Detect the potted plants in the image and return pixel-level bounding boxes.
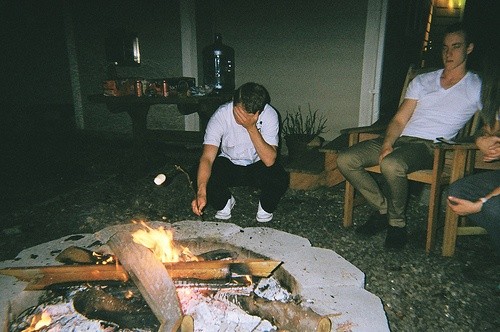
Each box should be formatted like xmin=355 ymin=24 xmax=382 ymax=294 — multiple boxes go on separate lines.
xmin=281 ymin=105 xmax=328 ymax=165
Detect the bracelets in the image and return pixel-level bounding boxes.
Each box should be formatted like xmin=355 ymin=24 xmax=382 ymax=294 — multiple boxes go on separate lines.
xmin=479 ymin=197 xmax=488 ymax=203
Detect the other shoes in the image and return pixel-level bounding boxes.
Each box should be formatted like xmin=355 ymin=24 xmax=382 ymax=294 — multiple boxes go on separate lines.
xmin=215 ymin=195 xmax=235 ymax=219
xmin=357 ymin=213 xmax=388 ymax=233
xmin=385 ymin=224 xmax=406 ymax=250
xmin=256 ymin=200 xmax=273 ymax=222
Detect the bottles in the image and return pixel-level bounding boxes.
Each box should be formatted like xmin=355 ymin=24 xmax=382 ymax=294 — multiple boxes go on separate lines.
xmin=203 ymin=33 xmax=235 ymax=94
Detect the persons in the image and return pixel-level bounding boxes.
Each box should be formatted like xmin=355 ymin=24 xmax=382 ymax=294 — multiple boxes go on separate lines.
xmin=446 ymin=107 xmax=500 ymax=248
xmin=191 ymin=83 xmax=290 ymax=222
xmin=336 ymin=20 xmax=500 ymax=250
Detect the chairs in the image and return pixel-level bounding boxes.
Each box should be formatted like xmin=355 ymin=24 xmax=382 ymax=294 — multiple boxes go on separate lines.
xmin=342 ymin=48 xmax=500 ymax=257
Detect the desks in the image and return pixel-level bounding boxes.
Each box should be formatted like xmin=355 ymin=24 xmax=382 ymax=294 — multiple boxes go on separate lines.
xmin=86 ymin=94 xmax=231 ymax=173
